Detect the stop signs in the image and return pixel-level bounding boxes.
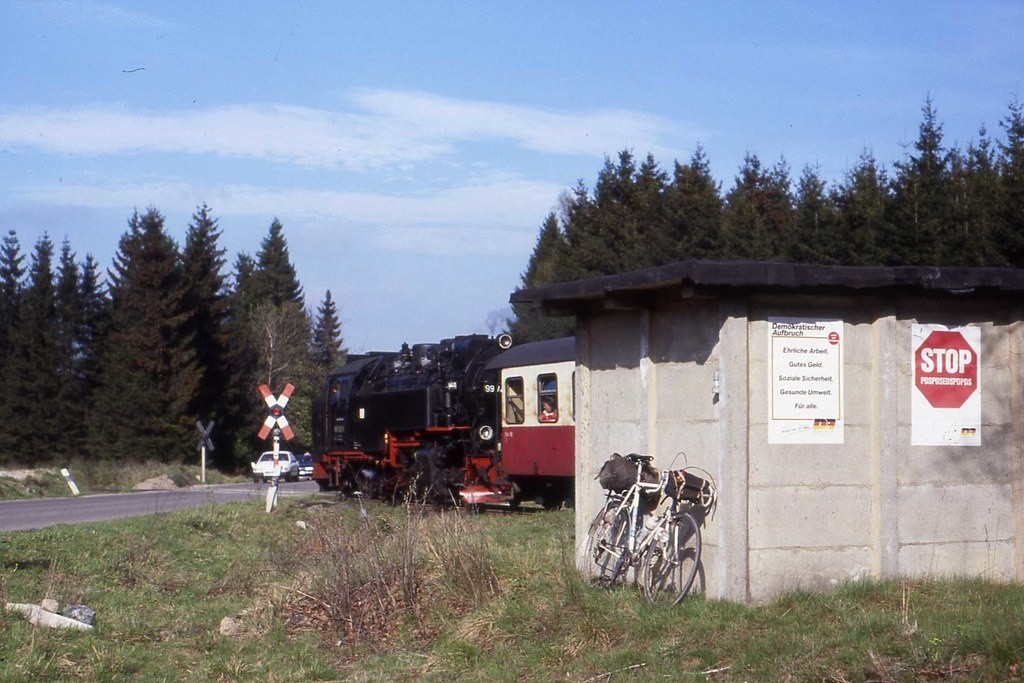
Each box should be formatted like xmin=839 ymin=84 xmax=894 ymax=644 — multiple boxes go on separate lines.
xmin=913 ymin=323 xmax=979 ymax=410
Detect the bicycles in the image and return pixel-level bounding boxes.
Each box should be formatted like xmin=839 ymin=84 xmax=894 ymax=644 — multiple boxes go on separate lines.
xmin=582 ymin=454 xmax=716 ymax=611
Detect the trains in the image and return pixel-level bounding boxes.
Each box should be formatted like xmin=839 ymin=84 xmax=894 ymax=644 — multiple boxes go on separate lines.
xmin=310 ymin=333 xmax=576 ymax=510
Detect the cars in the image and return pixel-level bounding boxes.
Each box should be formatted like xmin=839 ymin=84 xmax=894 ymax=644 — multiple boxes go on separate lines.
xmin=252 ymin=450 xmax=301 ymax=484
xmin=294 ymin=454 xmax=313 ymax=481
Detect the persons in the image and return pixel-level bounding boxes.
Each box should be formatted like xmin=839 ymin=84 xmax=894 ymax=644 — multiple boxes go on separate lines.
xmin=538 ymin=396 xmax=557 ymax=423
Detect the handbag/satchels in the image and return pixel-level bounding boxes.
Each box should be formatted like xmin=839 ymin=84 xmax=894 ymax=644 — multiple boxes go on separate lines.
xmin=665 ymin=470 xmax=714 ymax=508
xmin=594 ymin=453 xmax=661 ymax=506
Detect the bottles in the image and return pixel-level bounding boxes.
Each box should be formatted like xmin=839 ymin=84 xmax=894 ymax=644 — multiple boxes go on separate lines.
xmin=636 ymin=514 xmax=657 ymax=543
xmin=657 ymin=526 xmax=670 ymax=542
